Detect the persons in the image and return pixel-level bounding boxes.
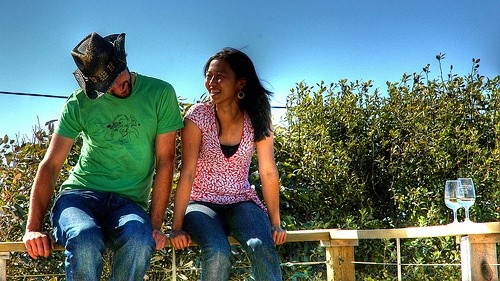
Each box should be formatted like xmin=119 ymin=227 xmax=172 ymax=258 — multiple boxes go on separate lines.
xmin=169 ymin=46 xmax=287 ymax=281
xmin=22 ymin=32 xmax=184 ymax=281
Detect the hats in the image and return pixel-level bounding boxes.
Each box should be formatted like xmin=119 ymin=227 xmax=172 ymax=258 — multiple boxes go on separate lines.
xmin=71 ymin=32 xmax=126 ymax=100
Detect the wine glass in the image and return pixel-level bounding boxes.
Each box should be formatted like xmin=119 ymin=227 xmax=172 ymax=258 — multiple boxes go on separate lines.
xmin=457 ymin=178 xmax=477 ymax=223
xmin=445 ymin=179 xmax=465 ymax=225
